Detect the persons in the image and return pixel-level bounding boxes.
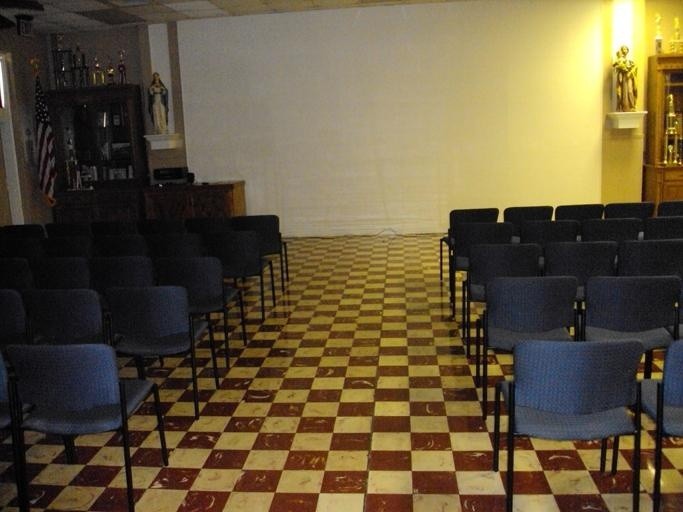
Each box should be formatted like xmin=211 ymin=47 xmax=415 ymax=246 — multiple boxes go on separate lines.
xmin=148 ymin=72 xmax=169 ymax=133
xmin=611 ymin=50 xmax=624 ymax=110
xmin=620 ymin=47 xmax=637 ymax=111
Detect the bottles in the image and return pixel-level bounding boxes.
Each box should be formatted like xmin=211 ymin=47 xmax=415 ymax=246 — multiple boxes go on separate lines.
xmin=70 ymin=49 xmax=126 ymax=88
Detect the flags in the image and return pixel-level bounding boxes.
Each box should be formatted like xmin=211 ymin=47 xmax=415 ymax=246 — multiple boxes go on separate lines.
xmin=28 ymin=69 xmax=59 ymax=208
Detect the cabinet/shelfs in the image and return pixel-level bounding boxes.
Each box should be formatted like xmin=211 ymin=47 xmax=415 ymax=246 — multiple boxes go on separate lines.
xmin=141 ymin=180 xmax=246 ymax=220
xmin=645 ymin=54 xmax=683 ymax=218
xmin=45 ymin=82 xmax=149 ymax=222
